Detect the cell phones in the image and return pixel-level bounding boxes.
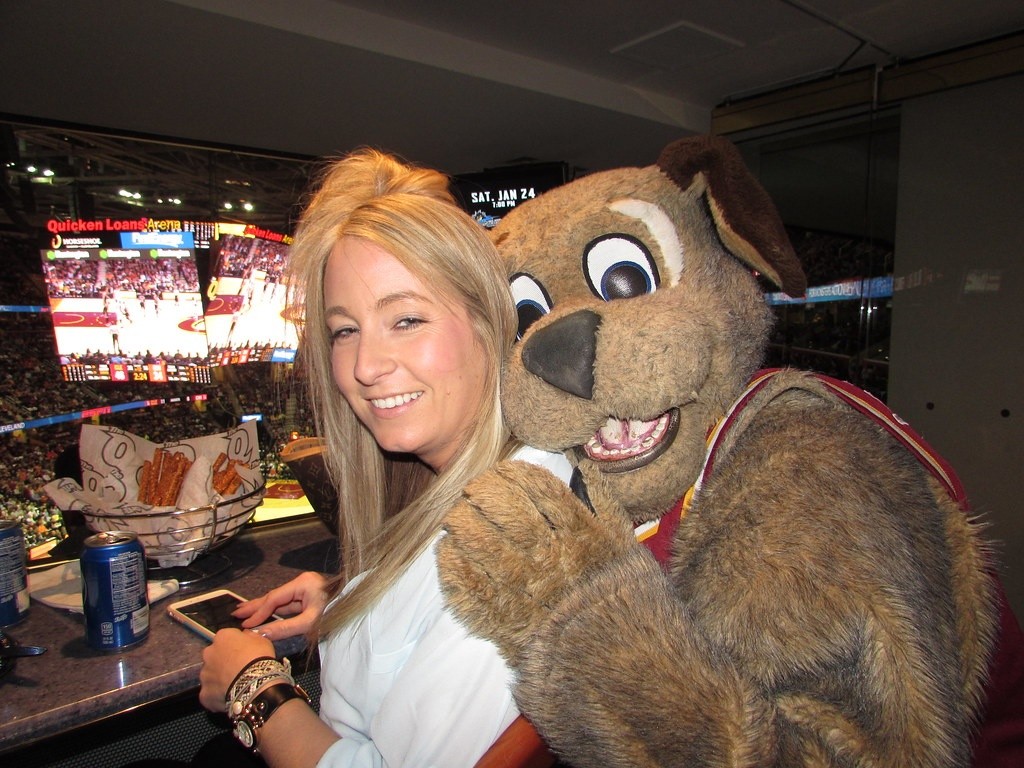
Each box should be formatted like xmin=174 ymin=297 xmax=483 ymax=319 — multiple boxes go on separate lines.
xmin=166 ymin=590 xmax=284 ymax=643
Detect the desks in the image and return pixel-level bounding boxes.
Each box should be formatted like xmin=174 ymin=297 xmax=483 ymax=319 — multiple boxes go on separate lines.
xmin=0 ymin=519 xmax=347 ymax=758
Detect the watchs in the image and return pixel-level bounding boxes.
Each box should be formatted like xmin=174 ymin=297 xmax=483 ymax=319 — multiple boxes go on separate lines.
xmin=233 ymin=684 xmax=311 ymax=753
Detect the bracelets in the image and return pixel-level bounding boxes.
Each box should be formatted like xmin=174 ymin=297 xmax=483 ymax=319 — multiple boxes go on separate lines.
xmin=225 ymin=657 xmax=295 ymax=716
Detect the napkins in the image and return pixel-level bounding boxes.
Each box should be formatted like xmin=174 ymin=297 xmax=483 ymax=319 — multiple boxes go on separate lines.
xmin=24 ymin=558 xmax=180 ymax=615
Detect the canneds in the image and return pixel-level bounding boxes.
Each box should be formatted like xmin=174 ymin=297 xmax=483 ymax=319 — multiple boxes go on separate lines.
xmin=80 ymin=530 xmax=151 ymax=651
xmin=0 ymin=519 xmax=32 ymax=629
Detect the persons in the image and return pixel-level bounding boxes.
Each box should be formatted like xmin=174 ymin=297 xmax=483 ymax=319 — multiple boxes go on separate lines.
xmin=47 ymin=257 xmax=208 ymax=366
xmin=0 ymin=283 xmax=317 ymax=548
xmin=208 ymin=233 xmax=292 ymax=351
xmin=200 ymin=152 xmax=574 ymax=768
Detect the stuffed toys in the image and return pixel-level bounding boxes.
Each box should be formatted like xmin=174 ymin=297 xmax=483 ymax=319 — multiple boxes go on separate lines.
xmin=433 ymin=134 xmax=1024 ymax=768
